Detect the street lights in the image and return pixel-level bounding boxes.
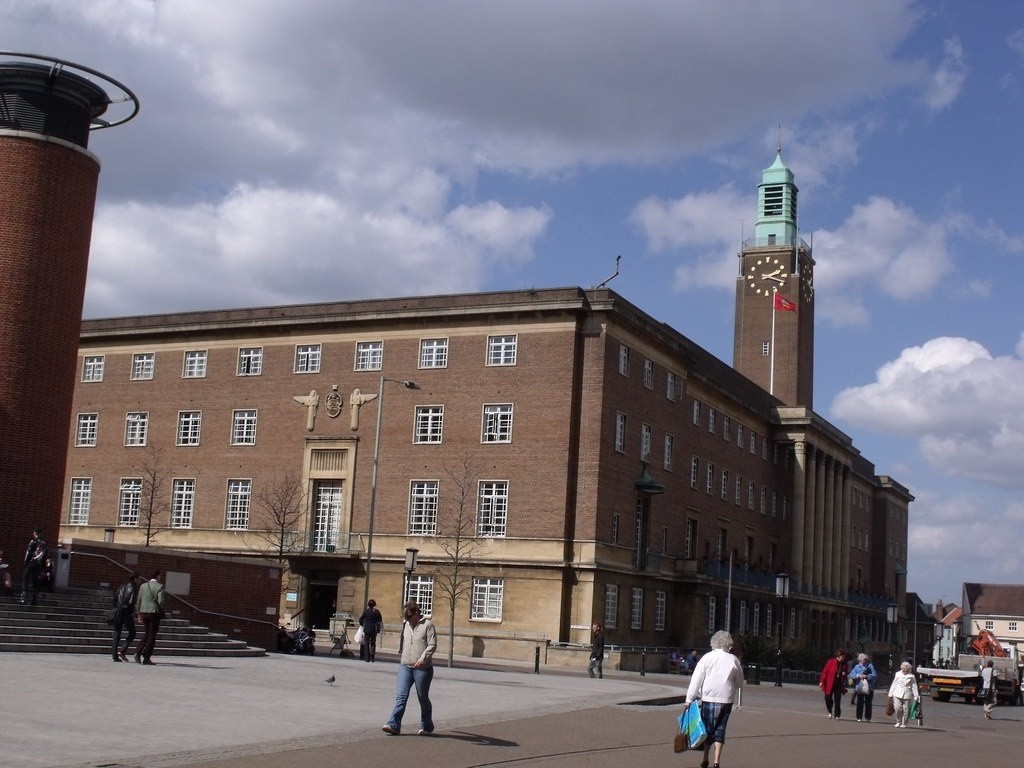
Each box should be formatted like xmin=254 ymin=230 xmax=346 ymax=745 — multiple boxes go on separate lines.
xmin=774 ymin=573 xmax=789 ymax=687
xmin=398 ymin=547 xmax=419 ymax=654
xmin=936 ymin=622 xmax=944 ymax=663
xmin=363 ymin=375 xmax=421 ymax=608
xmin=886 ymin=603 xmax=898 ymax=691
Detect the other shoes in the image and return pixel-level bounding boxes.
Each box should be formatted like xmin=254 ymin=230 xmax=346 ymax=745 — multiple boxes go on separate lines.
xmin=985 ymin=711 xmax=992 ymax=719
xmin=713 ymin=763 xmax=720 ymax=768
xmin=382 ymin=725 xmax=400 ymax=736
xmin=866 ymin=719 xmax=870 ymax=722
xmin=118 ymin=650 xmax=129 ymax=662
xmin=835 ymin=716 xmax=840 ymax=720
xmin=111 ymin=658 xmax=123 ymax=663
xmin=829 ymin=713 xmax=832 ymax=718
xmin=418 ymin=728 xmax=433 ymax=735
xmin=900 ymin=724 xmax=907 ymax=728
xmin=134 ymin=653 xmax=141 ymax=663
xmin=700 ymin=761 xmax=709 ymax=768
xmin=142 ymin=658 xmax=157 ymax=665
xmin=895 ymin=722 xmax=901 ymax=727
xmin=857 ymin=719 xmax=862 ymax=722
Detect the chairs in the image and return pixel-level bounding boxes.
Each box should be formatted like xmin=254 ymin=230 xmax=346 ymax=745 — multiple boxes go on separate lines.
xmin=667 ymin=650 xmax=689 ymax=675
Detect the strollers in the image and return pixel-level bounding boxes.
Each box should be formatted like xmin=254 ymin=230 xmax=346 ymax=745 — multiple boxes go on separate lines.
xmin=360 ymin=631 xmax=365 ymax=660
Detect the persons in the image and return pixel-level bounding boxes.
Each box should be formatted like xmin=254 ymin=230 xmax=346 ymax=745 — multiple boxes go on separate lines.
xmin=360 ymin=599 xmax=382 ymax=662
xmin=886 ymin=661 xmax=919 ymax=728
xmin=382 ymin=602 xmax=437 ymax=736
xmin=819 ymin=650 xmax=848 ymax=720
xmin=849 ymin=653 xmax=876 ymax=723
xmin=135 ymin=569 xmax=166 ymax=665
xmin=113 ymin=573 xmax=138 ymax=662
xmin=672 ymin=647 xmax=698 ymax=675
xmin=982 ymin=660 xmax=998 ymax=720
xmin=586 ymin=623 xmax=604 ymax=679
xmin=0 ymin=528 xmax=56 ymax=605
xmin=685 ymin=631 xmax=743 ymax=768
xmin=851 ymin=658 xmax=867 ymax=705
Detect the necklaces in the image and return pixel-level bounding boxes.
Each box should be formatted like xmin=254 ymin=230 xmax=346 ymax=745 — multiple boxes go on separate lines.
xmin=837 ymin=664 xmax=841 ymax=677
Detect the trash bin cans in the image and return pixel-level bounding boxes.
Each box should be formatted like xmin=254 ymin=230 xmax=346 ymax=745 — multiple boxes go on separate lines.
xmin=746 ymin=663 xmax=761 ymax=684
xmin=48 ymin=549 xmax=70 ymax=588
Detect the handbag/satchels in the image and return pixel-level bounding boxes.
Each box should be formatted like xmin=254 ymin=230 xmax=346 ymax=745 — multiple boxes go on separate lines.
xmin=677 ymin=700 xmax=708 ymax=750
xmin=106 ymin=606 xmax=123 ymax=626
xmin=153 ymin=599 xmax=166 ymax=620
xmin=886 ymin=697 xmax=894 ymax=716
xmin=673 ymin=734 xmax=686 ymax=753
xmin=856 ymin=678 xmax=869 ymax=694
xmin=977 ymin=688 xmax=992 ymax=704
xmin=355 ymin=626 xmax=366 ymax=645
xmin=908 ymin=700 xmax=923 ymax=719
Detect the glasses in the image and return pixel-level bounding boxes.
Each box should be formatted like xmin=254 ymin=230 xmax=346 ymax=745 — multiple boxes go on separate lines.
xmin=400 ymin=612 xmax=416 ymax=620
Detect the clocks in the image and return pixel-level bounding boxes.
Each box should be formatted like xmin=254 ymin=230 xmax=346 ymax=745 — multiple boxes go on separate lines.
xmin=747 ymin=256 xmax=788 ymax=296
xmin=802 ymin=267 xmax=815 ymax=304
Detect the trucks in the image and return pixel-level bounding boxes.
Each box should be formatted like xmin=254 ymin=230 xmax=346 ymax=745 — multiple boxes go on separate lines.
xmin=916 ymin=644 xmax=1024 ymax=706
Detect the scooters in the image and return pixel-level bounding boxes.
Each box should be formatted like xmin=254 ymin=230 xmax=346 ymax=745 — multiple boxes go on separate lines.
xmin=275 ymin=622 xmax=316 ymax=654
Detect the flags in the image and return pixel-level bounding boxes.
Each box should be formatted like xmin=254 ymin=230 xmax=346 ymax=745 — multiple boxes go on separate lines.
xmin=775 ymin=292 xmax=797 ymax=314
